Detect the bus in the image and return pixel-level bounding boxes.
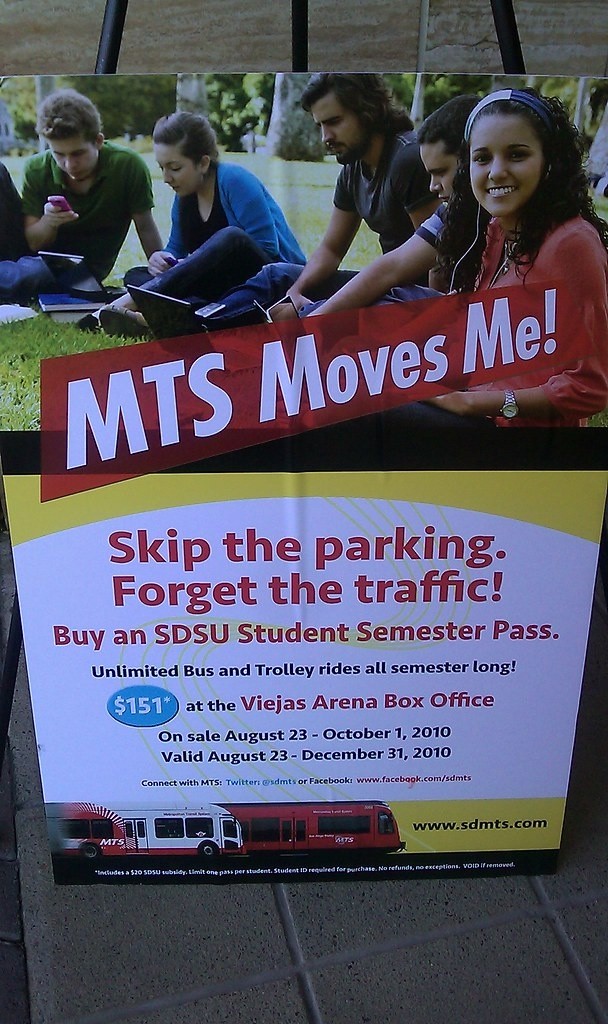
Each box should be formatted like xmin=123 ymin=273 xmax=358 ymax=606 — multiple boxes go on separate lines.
xmin=47 ymin=800 xmax=408 ymax=868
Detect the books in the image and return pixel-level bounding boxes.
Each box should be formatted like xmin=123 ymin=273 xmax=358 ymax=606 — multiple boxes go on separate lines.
xmin=38 ymin=293 xmax=105 ymax=325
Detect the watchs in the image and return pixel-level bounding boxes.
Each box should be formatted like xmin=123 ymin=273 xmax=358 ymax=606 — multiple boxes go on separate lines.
xmin=498 ymin=389 xmax=519 ymax=420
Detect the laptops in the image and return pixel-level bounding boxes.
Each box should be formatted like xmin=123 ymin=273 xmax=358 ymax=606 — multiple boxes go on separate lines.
xmin=38 ymin=251 xmax=127 ymax=301
xmin=127 ymin=284 xmax=265 ymax=376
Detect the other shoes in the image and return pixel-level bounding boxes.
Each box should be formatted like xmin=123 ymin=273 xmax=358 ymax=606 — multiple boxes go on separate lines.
xmin=99 ymin=304 xmax=152 ymax=340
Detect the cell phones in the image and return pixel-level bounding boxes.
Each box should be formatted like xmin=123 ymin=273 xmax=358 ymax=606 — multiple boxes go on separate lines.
xmin=253 ymin=299 xmax=267 ymax=323
xmin=195 ymin=302 xmax=226 ymax=317
xmin=47 ymin=196 xmax=72 ymax=212
xmin=266 ymin=294 xmax=300 ymax=324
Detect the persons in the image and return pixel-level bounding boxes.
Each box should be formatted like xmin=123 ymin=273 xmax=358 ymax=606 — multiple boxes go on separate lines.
xmin=76 ymin=112 xmax=307 ymax=335
xmin=0 ymin=90 xmax=163 ymax=302
xmin=204 ymin=74 xmax=608 ymax=428
